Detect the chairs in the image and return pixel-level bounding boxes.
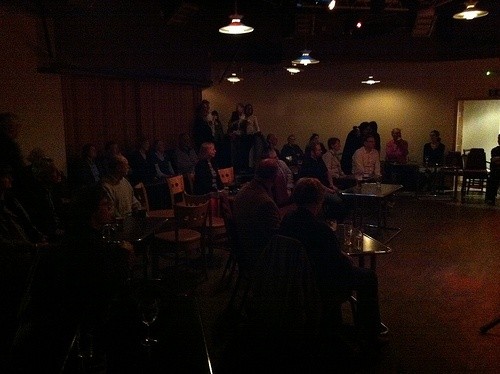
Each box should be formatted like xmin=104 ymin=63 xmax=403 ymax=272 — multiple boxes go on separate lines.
xmin=0 ymin=149 xmax=500 ymax=374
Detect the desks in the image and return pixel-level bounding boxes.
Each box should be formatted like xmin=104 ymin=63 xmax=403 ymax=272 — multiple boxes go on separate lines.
xmin=58 ymin=285 xmax=214 ymax=374
xmin=339 ymin=184 xmax=403 ymax=245
xmin=334 ymin=222 xmax=393 ymax=336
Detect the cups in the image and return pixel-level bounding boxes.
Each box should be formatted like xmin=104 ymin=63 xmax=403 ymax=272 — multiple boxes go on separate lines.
xmin=376 ymin=181 xmax=381 ymax=195
xmin=228 ymin=179 xmax=237 ymax=196
xmin=343 ymin=220 xmax=353 ymax=246
xmin=352 ymin=228 xmax=363 ymax=252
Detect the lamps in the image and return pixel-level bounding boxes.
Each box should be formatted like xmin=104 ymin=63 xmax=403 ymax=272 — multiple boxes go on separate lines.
xmin=291 ymin=41 xmax=320 ymax=65
xmin=452 ymin=0 xmax=489 ymax=21
xmin=219 ymin=0 xmax=254 ymax=34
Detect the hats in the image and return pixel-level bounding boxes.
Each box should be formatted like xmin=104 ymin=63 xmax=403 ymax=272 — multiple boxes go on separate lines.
xmin=110 ymin=155 xmax=126 ymax=173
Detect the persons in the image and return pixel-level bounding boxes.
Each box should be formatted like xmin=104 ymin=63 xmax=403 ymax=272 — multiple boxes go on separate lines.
xmin=352 ymin=134 xmax=389 ymax=207
xmin=193 ymin=141 xmax=224 ymax=195
xmin=423 ymin=130 xmax=450 ymax=168
xmin=385 ymin=128 xmax=409 ymax=165
xmin=0 ymin=99 xmax=500 ymax=335
